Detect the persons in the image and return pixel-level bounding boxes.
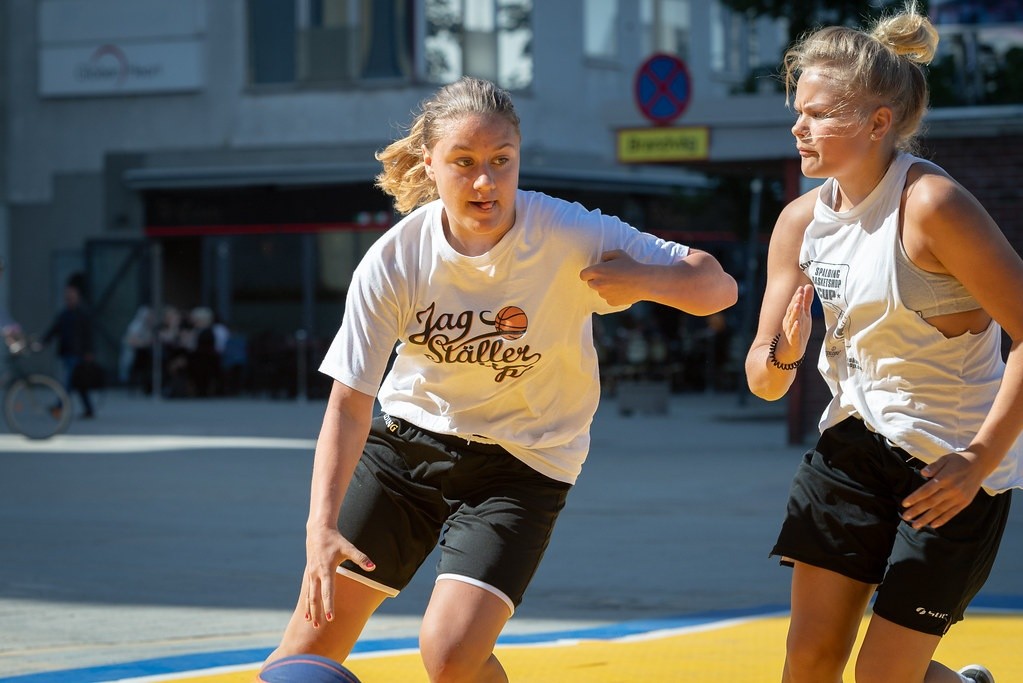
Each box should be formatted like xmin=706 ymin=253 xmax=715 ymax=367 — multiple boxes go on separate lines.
xmin=32 ymin=278 xmax=97 ymax=418
xmin=119 ymin=304 xmax=223 ymax=401
xmin=745 ymin=0 xmax=1023 ymax=683
xmin=263 ymin=77 xmax=738 ymax=683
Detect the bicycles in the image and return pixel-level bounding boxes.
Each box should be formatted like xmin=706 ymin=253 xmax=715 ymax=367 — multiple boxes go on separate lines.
xmin=0 ymin=335 xmax=72 ymax=441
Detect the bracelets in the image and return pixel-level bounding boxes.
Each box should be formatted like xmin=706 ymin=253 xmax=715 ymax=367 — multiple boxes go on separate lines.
xmin=769 ymin=333 xmax=805 ymax=370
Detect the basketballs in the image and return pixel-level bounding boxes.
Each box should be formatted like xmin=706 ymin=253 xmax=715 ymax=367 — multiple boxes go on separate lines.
xmin=257 ymin=654 xmax=364 ymax=683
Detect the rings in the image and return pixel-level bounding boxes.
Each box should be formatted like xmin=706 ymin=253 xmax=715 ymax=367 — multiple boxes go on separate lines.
xmin=933 ymin=477 xmax=943 ymax=489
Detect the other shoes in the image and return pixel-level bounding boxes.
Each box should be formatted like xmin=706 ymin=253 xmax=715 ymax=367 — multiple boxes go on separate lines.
xmin=960 ymin=665 xmax=994 ymax=683
